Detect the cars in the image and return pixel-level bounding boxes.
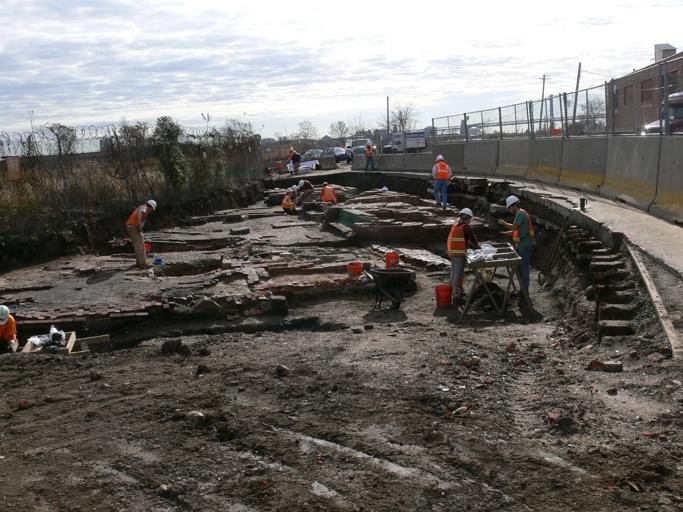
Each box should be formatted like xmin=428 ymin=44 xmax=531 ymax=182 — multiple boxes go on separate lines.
xmin=324 ymin=146 xmax=346 ymax=164
xmin=300 ymin=149 xmax=324 ymax=163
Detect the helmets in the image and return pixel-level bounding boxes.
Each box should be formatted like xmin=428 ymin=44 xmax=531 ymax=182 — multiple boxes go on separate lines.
xmin=435 ymin=155 xmax=443 ymax=161
xmin=505 ymin=195 xmax=519 ymax=208
xmin=459 ymin=208 xmax=472 ymax=216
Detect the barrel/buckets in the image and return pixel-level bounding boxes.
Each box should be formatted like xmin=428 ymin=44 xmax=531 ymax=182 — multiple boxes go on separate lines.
xmin=434 ymin=284 xmax=453 ymax=308
xmin=386 ymin=251 xmax=399 ymax=270
xmin=347 ymin=261 xmax=363 ymax=277
xmin=144 ymin=243 xmax=152 ymax=260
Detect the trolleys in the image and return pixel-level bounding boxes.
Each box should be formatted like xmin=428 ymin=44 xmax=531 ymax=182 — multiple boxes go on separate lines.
xmin=364 ymin=266 xmax=416 ymax=311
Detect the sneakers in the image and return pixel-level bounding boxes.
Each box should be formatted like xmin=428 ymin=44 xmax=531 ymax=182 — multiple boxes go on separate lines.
xmin=140 ymin=265 xmax=153 ymax=269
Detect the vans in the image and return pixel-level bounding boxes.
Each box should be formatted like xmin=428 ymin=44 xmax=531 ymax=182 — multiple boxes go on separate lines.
xmin=346 ymin=138 xmax=371 ymax=159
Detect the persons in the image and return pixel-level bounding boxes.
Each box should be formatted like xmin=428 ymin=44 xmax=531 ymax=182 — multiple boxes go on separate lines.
xmin=281 ymin=180 xmax=336 ymax=214
xmin=447 ymin=208 xmax=478 ymax=304
xmin=432 ymin=155 xmax=453 ymax=208
xmin=498 ymin=195 xmax=536 ymax=298
xmin=365 ymin=144 xmax=377 ymax=170
xmin=0 ymin=305 xmax=18 ymax=351
xmin=288 ymin=147 xmax=301 ymax=175
xmin=127 ymin=200 xmax=158 ymax=268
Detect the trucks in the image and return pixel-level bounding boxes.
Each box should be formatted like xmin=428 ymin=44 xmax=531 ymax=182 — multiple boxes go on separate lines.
xmin=382 ymin=131 xmax=425 ymax=154
xmin=642 ymin=91 xmax=683 ymax=135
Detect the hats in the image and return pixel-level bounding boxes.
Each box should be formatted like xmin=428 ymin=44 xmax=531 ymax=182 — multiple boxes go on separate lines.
xmin=287 ymin=147 xmax=294 ymax=150
xmin=286 ymin=180 xmax=303 ymax=192
xmin=147 ymin=199 xmax=157 ymax=212
xmin=0 ymin=305 xmax=10 ymax=326
